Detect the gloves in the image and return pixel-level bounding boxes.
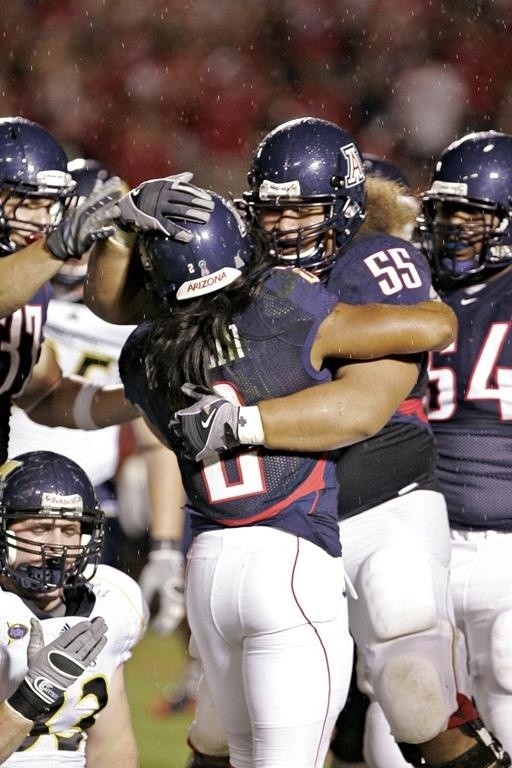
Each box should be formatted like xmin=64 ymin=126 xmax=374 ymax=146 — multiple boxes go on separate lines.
xmin=23 ymin=616 xmax=108 ymax=706
xmin=115 ymin=171 xmax=215 ymax=243
xmin=139 ymin=549 xmax=187 ymax=639
xmin=45 ymin=171 xmax=121 ymax=263
xmin=167 ymin=381 xmax=266 ymax=462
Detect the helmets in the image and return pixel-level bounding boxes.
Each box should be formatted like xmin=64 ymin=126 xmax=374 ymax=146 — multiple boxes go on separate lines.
xmin=0 ymin=450 xmax=108 ymax=594
xmin=48 ymin=168 xmax=104 ymax=289
xmin=415 ymin=131 xmax=511 ymax=282
xmin=136 ymin=188 xmax=253 ymax=303
xmin=359 ymin=153 xmax=412 ymax=188
xmin=227 ymin=116 xmax=370 ymax=275
xmin=0 ymin=116 xmax=80 ymax=259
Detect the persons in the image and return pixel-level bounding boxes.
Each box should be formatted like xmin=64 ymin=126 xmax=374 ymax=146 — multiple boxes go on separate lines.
xmin=0 ymin=451 xmax=149 ymax=768
xmin=0 ymin=116 xmax=142 ymax=464
xmin=421 ymin=131 xmax=511 ymax=757
xmin=360 ymin=154 xmax=422 ymax=240
xmin=83 ymin=117 xmax=512 ymax=768
xmin=119 ymin=189 xmax=459 ymax=768
xmin=0 ymin=158 xmax=191 ymax=638
